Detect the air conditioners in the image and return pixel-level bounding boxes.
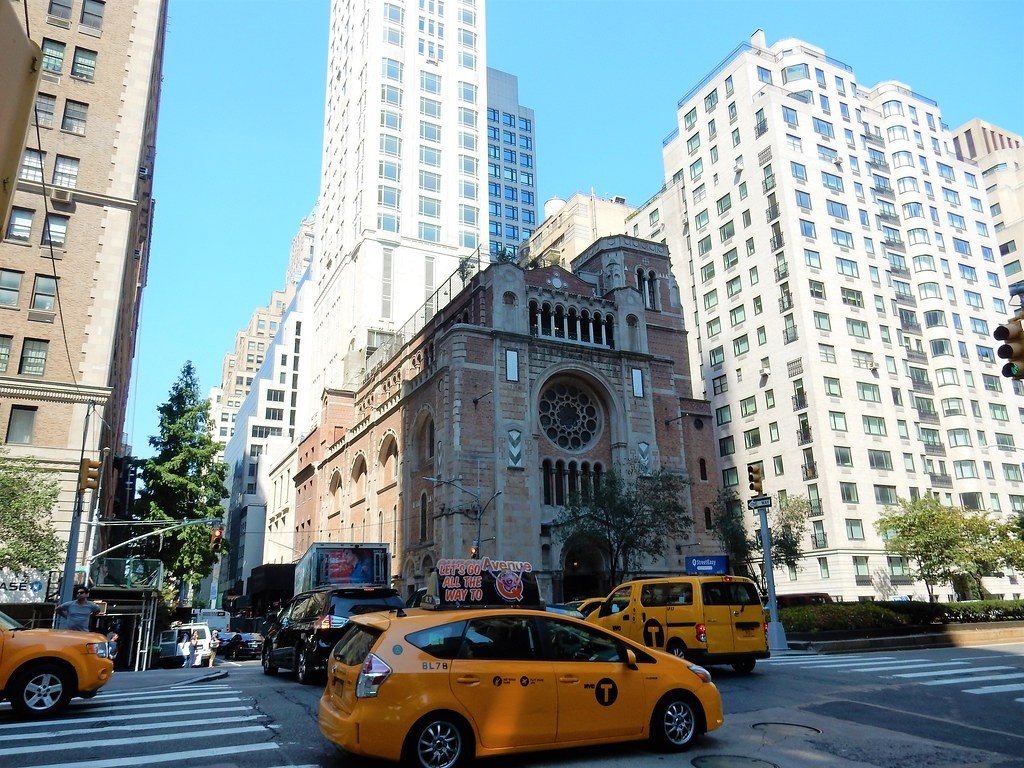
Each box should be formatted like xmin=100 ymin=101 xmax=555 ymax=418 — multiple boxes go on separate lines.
xmin=50 ymin=188 xmax=73 ymax=205
xmin=140 ymin=166 xmax=148 ymax=181
xmin=760 ymin=368 xmax=770 ymax=375
xmin=868 ymin=362 xmax=880 ymax=370
xmin=135 ymin=250 xmax=141 ymax=259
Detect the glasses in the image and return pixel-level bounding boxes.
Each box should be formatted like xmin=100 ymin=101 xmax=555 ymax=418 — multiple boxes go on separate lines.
xmin=76 ymin=591 xmax=84 ymax=594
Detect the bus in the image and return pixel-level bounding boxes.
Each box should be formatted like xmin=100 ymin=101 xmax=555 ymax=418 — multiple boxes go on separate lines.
xmin=196 ymin=610 xmax=231 ymax=633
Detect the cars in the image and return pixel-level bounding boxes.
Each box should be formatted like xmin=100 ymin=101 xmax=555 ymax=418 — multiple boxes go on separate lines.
xmin=546 ymin=593 xmax=669 ymax=622
xmin=224 ymin=632 xmax=265 ymax=660
xmin=214 ymin=630 xmax=238 ymax=655
xmin=315 ymin=556 xmax=726 ymax=768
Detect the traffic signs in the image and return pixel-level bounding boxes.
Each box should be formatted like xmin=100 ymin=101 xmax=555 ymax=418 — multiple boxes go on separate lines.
xmin=747 ymin=497 xmax=772 ymax=510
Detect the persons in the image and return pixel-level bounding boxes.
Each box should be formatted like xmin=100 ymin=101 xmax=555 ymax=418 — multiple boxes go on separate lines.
xmin=107 ymin=633 xmax=119 ymax=673
xmin=176 ymin=630 xmax=222 ymax=669
xmin=342 ymin=548 xmax=375 ymax=584
xmin=54 ymin=587 xmax=101 ymax=633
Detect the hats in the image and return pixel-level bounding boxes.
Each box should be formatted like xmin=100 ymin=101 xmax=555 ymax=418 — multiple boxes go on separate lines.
xmin=213 ymin=630 xmax=218 ymax=634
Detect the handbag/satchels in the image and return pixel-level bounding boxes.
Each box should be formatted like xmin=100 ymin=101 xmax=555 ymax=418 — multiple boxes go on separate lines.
xmin=210 ymin=643 xmax=220 ymax=648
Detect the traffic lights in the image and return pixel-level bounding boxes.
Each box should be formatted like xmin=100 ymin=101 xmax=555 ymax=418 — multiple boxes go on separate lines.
xmin=209 ymin=528 xmax=223 ymax=554
xmin=992 ymin=317 xmax=1024 ymax=381
xmin=80 ymin=457 xmax=100 ymax=491
xmin=470 ymin=546 xmax=479 ymax=559
xmin=747 ymin=464 xmax=764 ymax=493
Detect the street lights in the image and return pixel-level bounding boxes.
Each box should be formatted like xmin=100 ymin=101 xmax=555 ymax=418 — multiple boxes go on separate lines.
xmin=422 ymin=459 xmax=502 ymax=560
xmin=78 ymin=446 xmax=111 ymax=588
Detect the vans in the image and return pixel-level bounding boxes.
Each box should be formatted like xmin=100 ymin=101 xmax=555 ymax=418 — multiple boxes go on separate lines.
xmin=760 ymin=592 xmax=833 ymax=609
xmin=583 ymin=554 xmax=771 ymax=674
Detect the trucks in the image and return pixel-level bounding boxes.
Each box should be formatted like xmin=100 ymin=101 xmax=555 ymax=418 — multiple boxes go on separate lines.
xmin=292 ymin=542 xmax=394 ymax=598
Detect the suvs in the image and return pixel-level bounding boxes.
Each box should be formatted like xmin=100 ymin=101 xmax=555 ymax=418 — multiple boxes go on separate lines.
xmin=404 ymin=584 xmax=429 ymax=607
xmin=262 ymin=586 xmax=407 ymax=686
xmin=0 ymin=609 xmax=118 ymax=722
xmin=159 ymin=622 xmax=213 ymax=666
